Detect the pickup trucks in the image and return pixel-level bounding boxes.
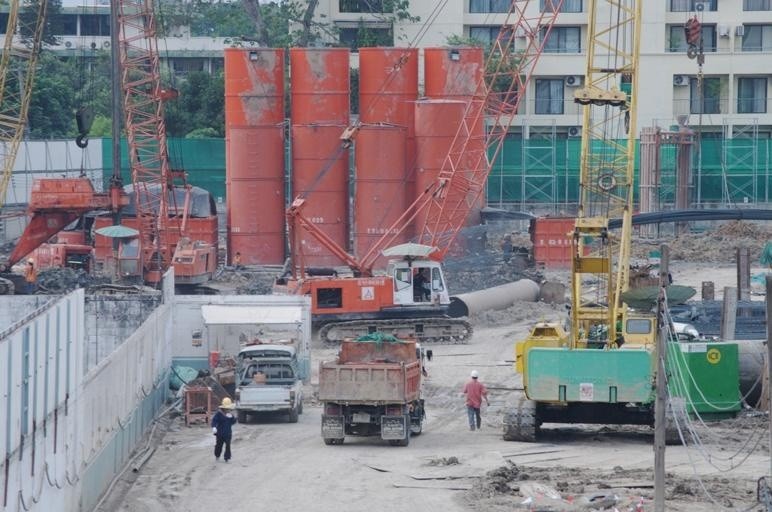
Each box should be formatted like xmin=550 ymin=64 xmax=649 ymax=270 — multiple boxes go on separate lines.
xmin=234 ymin=360 xmax=302 ymax=423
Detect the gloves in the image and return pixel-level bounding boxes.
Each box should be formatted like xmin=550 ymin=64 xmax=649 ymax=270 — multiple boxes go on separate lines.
xmin=212 ymin=427 xmax=217 ymax=433
xmin=226 ymin=413 xmax=233 ymax=418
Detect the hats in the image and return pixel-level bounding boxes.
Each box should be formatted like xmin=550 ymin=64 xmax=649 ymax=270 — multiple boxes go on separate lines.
xmin=217 ymin=397 xmax=236 ymax=410
xmin=471 ymin=370 xmax=479 ymax=377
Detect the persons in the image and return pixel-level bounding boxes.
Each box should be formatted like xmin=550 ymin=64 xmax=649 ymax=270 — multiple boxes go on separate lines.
xmin=278 ymin=252 xmax=294 ymax=278
xmin=23 ymin=256 xmax=38 ymax=295
xmin=413 ymin=267 xmax=430 ymax=302
xmin=211 ymin=396 xmax=238 ymax=463
xmin=463 ymin=368 xmax=491 ymax=431
xmin=232 ymin=251 xmax=248 ymax=272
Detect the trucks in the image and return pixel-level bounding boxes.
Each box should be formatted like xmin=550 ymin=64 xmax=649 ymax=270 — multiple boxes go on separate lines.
xmin=320 ymin=335 xmax=432 ymax=447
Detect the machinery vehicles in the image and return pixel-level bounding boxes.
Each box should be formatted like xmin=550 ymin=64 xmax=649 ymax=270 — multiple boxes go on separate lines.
xmin=503 ymin=0 xmax=742 ymax=444
xmin=0 ymin=0 xmax=217 ymax=295
xmin=272 ymin=0 xmax=564 ymax=347
